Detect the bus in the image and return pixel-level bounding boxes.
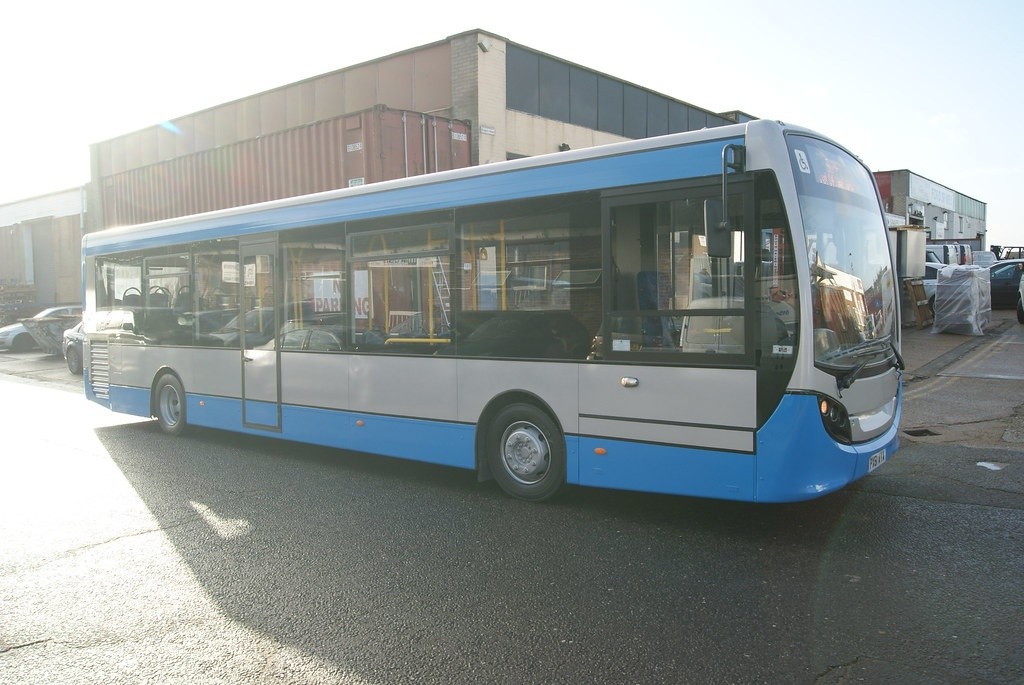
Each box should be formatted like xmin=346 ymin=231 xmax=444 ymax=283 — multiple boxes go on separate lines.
xmin=80 ymin=118 xmax=907 ymax=506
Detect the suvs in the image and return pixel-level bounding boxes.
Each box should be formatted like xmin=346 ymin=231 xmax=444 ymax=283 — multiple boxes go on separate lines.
xmin=679 ymin=295 xmax=796 ymax=356
xmin=972 ymin=250 xmax=998 ymax=267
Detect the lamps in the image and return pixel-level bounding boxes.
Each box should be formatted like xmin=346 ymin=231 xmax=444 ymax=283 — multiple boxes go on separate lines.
xmin=476 ymin=39 xmax=494 ymax=54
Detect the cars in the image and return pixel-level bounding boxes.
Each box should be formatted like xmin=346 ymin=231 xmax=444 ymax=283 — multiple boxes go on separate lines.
xmin=96 ymin=305 xmax=590 ymax=361
xmin=923 ymin=261 xmax=951 ymax=317
xmin=0 ymin=305 xmax=82 ymax=352
xmin=61 ymin=320 xmax=83 ymax=376
xmin=587 ymin=308 xmax=680 ymax=363
xmin=977 ymin=259 xmax=1024 ymax=307
xmin=1013 ymin=263 xmax=1024 ymax=324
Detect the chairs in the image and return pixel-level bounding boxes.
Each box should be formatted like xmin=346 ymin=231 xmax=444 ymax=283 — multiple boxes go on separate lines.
xmin=121 ymin=285 xmax=204 ymax=332
xmin=361 ymin=322 xmax=450 ymax=346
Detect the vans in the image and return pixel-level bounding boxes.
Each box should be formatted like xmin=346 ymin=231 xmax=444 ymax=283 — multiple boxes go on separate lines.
xmin=926 ymin=242 xmax=972 ymax=264
